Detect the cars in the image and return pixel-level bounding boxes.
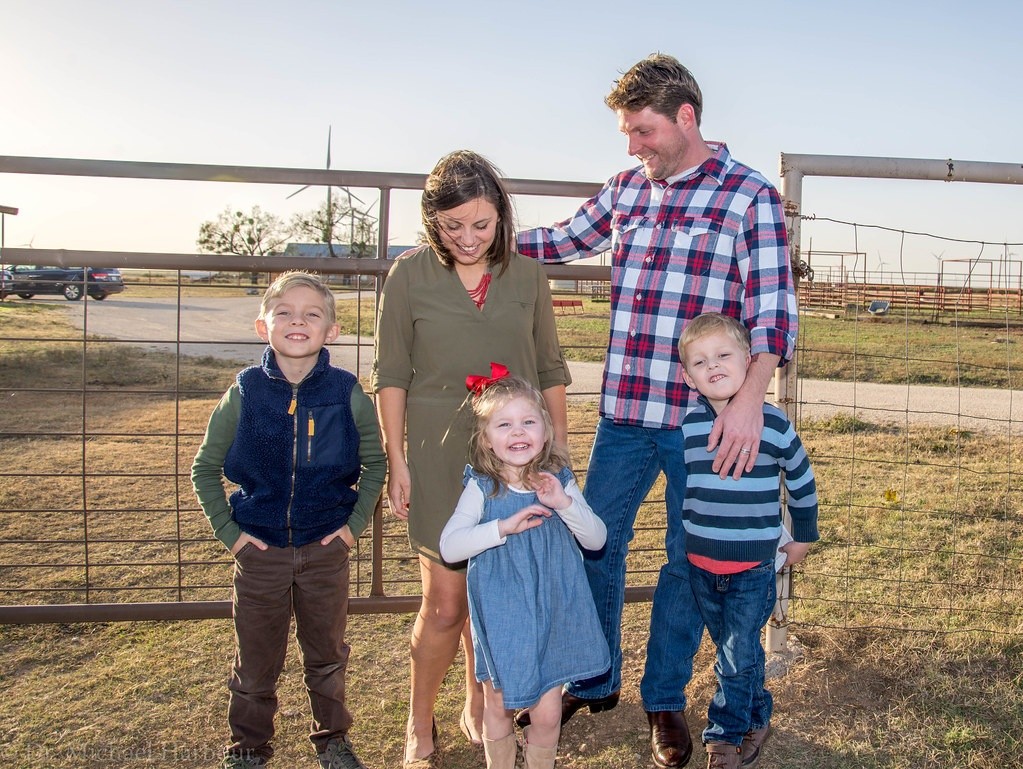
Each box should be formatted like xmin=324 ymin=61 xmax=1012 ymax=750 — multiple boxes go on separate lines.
xmin=0 ymin=270 xmax=14 ymax=300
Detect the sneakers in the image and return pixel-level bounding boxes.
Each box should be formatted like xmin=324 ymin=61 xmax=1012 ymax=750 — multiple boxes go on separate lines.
xmin=313 ymin=734 xmax=368 ymax=769
xmin=222 ymin=756 xmax=265 ymax=769
xmin=705 ymin=740 xmax=741 ymax=769
xmin=738 ymin=721 xmax=770 ymax=769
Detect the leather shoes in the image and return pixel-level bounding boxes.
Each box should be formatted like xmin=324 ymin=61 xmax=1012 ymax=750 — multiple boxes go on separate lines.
xmin=646 ymin=710 xmax=692 ymax=769
xmin=515 ymin=687 xmax=620 ymax=728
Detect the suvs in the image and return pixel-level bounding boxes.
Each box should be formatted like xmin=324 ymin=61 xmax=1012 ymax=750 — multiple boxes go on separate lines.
xmin=6 ymin=264 xmax=124 ymax=300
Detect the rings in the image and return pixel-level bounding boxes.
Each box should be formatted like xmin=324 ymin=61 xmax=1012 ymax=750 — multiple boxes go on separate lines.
xmin=741 ymin=449 xmax=751 ymax=454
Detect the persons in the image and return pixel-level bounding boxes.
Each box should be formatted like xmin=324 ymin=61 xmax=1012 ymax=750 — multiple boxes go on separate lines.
xmin=439 ymin=363 xmax=612 ymax=769
xmin=678 ymin=311 xmax=820 ymax=769
xmin=394 ymin=53 xmax=799 ymax=769
xmin=189 ymin=271 xmax=388 ymax=769
xmin=367 ymin=149 xmax=572 ymax=769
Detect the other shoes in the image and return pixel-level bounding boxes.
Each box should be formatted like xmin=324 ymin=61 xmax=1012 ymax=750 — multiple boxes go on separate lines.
xmin=459 ymin=709 xmax=480 ymax=744
xmin=403 ymin=715 xmax=436 ymax=769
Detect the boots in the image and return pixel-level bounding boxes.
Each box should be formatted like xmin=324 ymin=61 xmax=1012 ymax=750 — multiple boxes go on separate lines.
xmin=482 ymin=733 xmax=517 ymax=769
xmin=522 ymin=725 xmax=558 ymax=769
